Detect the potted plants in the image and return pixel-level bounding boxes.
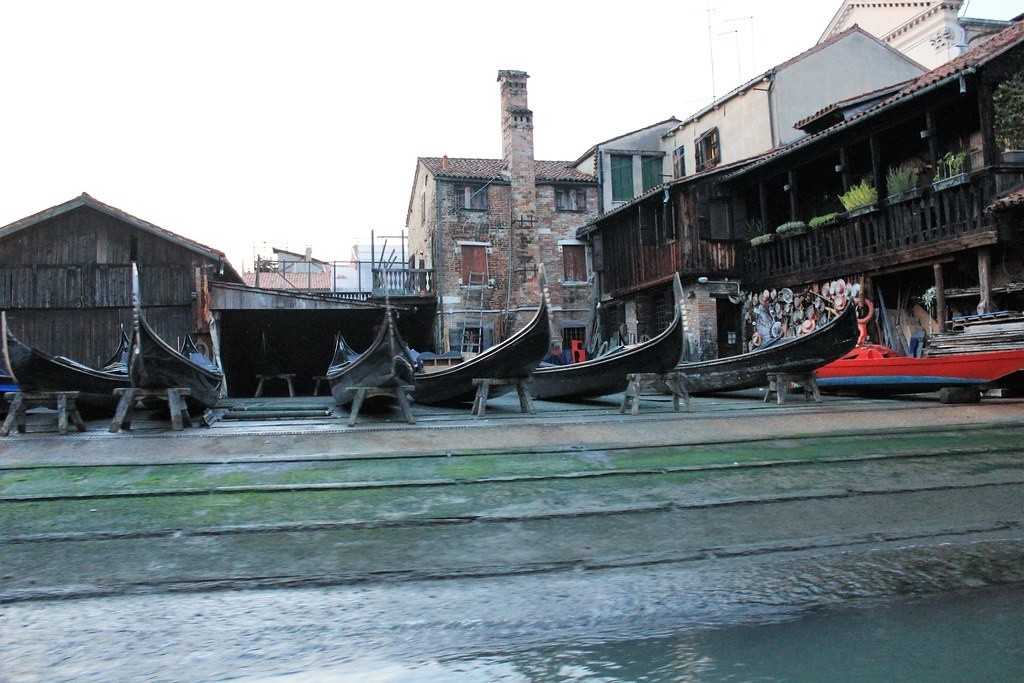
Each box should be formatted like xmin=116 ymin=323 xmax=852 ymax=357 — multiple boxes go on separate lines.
xmin=751 ymin=234 xmax=776 ymax=247
xmin=932 ymin=147 xmax=970 ymax=192
xmin=809 ymin=212 xmax=840 ymax=230
xmin=837 ymin=178 xmax=879 ymax=217
xmin=991 ymin=70 xmax=1024 ymax=163
xmin=776 ymin=220 xmax=810 ymax=240
xmin=886 ymin=165 xmax=924 ymax=204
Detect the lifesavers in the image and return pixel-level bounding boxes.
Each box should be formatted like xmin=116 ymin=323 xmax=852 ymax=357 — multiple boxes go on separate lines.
xmin=854 ymin=299 xmax=875 ymax=326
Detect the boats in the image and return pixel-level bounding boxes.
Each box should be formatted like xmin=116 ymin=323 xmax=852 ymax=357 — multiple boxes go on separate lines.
xmin=525 ymin=305 xmax=685 ymax=401
xmin=325 ymin=305 xmax=414 ymax=406
xmin=407 ymin=299 xmax=552 ymax=408
xmin=1 ymin=311 xmax=131 ymax=409
xmin=126 ymin=261 xmax=224 ymax=411
xmin=808 ymin=343 xmax=1024 ymax=391
xmin=641 ymin=296 xmax=860 ymax=397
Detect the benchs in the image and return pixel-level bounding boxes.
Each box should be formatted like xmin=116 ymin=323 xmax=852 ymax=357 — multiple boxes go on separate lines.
xmin=313 ymin=376 xmax=327 ymax=398
xmin=108 ymin=387 xmax=192 ymax=433
xmin=345 ymin=385 xmax=416 ymax=426
xmin=764 ymin=371 xmax=822 ymax=404
xmin=618 ymin=371 xmax=692 ymax=415
xmin=471 ymin=377 xmax=536 ymax=417
xmin=255 ymin=374 xmax=296 ymax=397
xmin=0 ymin=391 xmax=87 ymax=437
